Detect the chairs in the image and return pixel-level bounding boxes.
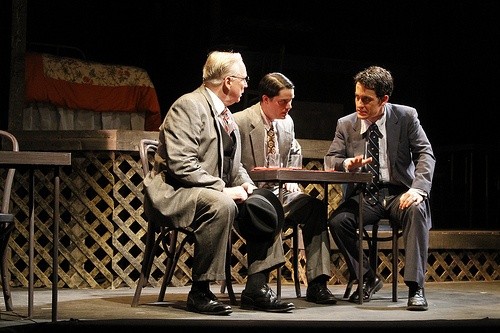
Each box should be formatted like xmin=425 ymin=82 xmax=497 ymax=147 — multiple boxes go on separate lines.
xmin=0 ymin=130 xmax=23 ymax=311
xmin=133 ymin=139 xmax=408 ymax=310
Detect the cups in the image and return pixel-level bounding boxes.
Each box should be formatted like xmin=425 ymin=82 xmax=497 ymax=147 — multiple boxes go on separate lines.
xmin=268 ymin=154 xmax=280 ymax=169
xmin=291 ymin=155 xmax=303 ymax=169
xmin=324 ymin=156 xmax=335 ymax=171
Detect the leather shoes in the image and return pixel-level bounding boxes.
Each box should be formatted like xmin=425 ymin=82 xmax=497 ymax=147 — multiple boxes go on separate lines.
xmin=186 ymin=291 xmax=233 ymax=315
xmin=407 ymin=287 xmax=428 ymax=311
xmin=241 ymin=282 xmax=295 ymax=312
xmin=349 ymin=271 xmax=383 ymax=303
xmin=305 ymin=283 xmax=338 ymax=304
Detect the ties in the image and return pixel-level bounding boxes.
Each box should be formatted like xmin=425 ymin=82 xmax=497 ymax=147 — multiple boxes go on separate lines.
xmin=222 ymin=105 xmax=237 ymax=138
xmin=361 ymin=123 xmax=382 ymax=206
xmin=262 ymin=125 xmax=280 ymax=190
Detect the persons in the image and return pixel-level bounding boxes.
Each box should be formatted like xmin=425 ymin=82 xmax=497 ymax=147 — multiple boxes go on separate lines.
xmin=228 ymin=73 xmax=338 ymax=304
xmin=325 ymin=67 xmax=436 ymax=311
xmin=146 ymin=51 xmax=294 ymax=314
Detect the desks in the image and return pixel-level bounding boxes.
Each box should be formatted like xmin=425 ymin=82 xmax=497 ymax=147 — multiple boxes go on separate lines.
xmin=249 ymin=168 xmax=375 ymax=304
xmin=1 ymin=152 xmax=72 ymax=327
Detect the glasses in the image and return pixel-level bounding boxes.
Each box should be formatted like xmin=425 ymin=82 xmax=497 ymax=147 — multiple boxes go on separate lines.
xmin=228 ymin=75 xmax=250 ymax=83
